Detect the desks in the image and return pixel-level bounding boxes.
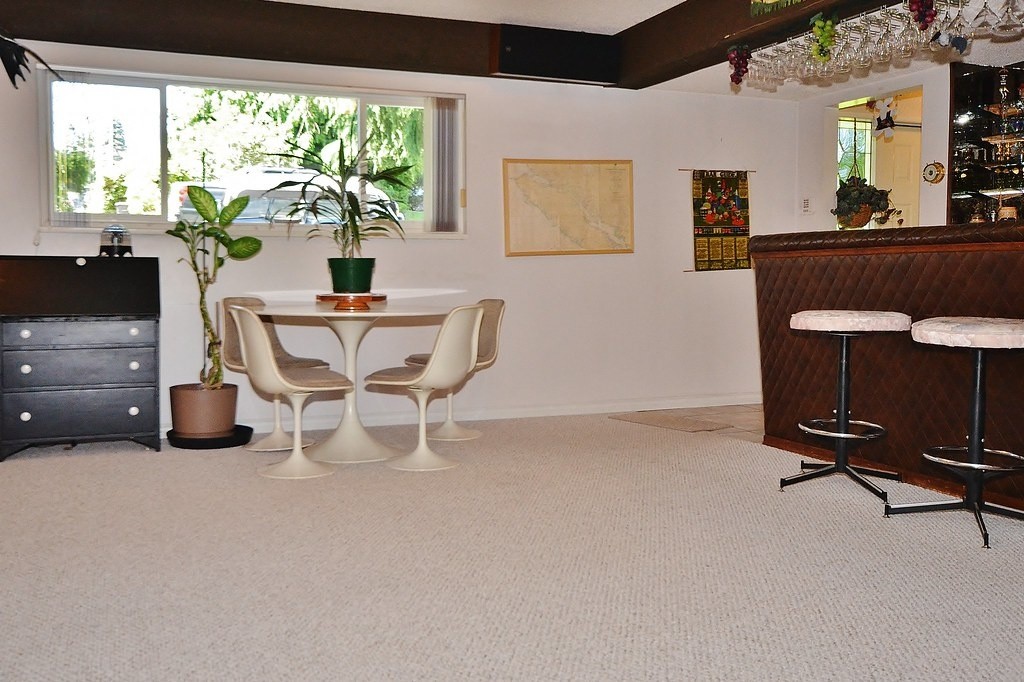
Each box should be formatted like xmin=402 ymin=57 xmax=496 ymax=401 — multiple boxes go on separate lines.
xmin=248 ymin=292 xmax=446 ymax=463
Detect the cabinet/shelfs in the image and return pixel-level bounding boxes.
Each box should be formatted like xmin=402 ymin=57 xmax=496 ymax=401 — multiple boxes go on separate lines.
xmin=1 ymin=311 xmax=160 ymax=441
xmin=957 ymin=105 xmax=1024 ymax=198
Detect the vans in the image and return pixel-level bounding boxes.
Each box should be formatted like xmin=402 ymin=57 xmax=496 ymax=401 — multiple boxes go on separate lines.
xmin=169 ymin=165 xmax=403 ymax=223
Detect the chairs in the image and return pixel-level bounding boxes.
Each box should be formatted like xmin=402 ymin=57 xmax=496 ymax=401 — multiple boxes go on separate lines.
xmin=222 ymin=296 xmax=330 ymax=451
xmin=405 ymin=288 xmax=506 ymax=441
xmin=229 ymin=304 xmax=353 ymax=480
xmin=365 ymin=304 xmax=487 ymax=470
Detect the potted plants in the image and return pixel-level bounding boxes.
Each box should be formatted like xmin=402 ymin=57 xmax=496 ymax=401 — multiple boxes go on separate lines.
xmin=165 ymin=184 xmax=264 ymax=435
xmin=830 ymin=171 xmax=904 ymax=228
xmin=257 ymin=131 xmax=417 ymax=293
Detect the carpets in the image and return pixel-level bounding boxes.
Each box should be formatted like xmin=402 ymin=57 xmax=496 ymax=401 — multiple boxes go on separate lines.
xmin=0 ymin=414 xmax=1022 ymax=682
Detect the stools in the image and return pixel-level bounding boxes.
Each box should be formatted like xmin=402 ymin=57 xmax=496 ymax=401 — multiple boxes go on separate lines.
xmin=778 ymin=310 xmax=909 ymax=505
xmin=886 ymin=315 xmax=1024 ymax=547
